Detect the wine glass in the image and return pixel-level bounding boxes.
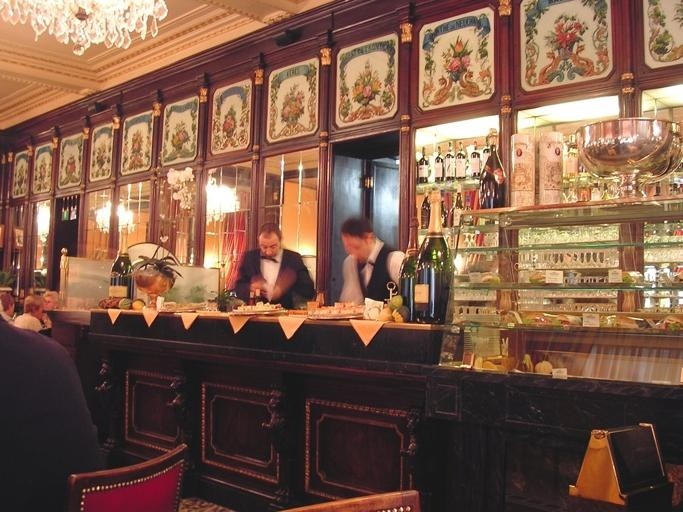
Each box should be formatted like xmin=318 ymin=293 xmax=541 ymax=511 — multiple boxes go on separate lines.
xmin=133 ymin=271 xmax=177 ymax=308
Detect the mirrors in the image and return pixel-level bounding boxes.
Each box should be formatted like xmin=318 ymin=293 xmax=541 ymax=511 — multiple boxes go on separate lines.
xmin=32 ymin=145 xmax=320 ymax=297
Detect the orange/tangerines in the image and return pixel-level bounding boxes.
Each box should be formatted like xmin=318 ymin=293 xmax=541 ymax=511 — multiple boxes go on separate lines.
xmin=118 ymin=298 xmax=130 ymax=309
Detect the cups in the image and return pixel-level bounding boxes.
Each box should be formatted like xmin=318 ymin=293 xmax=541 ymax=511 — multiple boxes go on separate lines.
xmin=517 ymin=225 xmax=619 ymax=313
xmin=644 ymin=221 xmax=682 ymax=313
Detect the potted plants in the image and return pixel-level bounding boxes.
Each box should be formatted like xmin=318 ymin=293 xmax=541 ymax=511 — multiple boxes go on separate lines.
xmin=128 ymin=239 xmax=185 ymax=310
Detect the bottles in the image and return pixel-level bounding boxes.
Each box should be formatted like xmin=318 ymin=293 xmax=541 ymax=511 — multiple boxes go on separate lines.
xmin=561 ymin=115 xmax=683 ymax=208
xmin=399 ymin=138 xmax=505 ymax=324
xmin=107 ymin=224 xmax=133 ymax=298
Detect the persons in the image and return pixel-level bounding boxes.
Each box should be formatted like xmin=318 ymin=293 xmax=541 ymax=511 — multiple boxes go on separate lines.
xmin=337 ymin=216 xmax=407 ymax=307
xmin=0 ymin=289 xmax=106 ymax=512
xmin=235 ymin=223 xmax=315 ymax=309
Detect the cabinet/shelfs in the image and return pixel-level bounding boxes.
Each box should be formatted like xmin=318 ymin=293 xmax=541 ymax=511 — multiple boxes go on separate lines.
xmin=407 ymin=103 xmax=682 ymax=330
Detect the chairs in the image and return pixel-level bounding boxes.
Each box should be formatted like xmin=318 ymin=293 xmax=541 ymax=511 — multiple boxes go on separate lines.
xmin=60 ymin=440 xmax=189 ymax=512
xmin=270 ymin=484 xmax=421 ymax=511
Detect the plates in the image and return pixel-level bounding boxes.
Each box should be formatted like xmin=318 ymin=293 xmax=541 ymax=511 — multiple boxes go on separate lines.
xmin=162 ymin=288 xmax=362 ymax=319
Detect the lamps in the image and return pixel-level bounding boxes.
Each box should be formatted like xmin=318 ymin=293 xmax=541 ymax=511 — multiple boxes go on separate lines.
xmin=0 ymin=0 xmax=168 ymax=59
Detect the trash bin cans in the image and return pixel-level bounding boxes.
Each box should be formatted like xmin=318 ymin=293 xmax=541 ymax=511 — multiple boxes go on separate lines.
xmin=568 ymin=423 xmax=674 ymax=512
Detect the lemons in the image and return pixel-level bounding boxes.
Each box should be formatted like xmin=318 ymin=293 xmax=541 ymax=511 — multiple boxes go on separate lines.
xmin=131 ymin=301 xmax=145 ymax=310
xmin=389 ymin=295 xmax=404 ymax=310
